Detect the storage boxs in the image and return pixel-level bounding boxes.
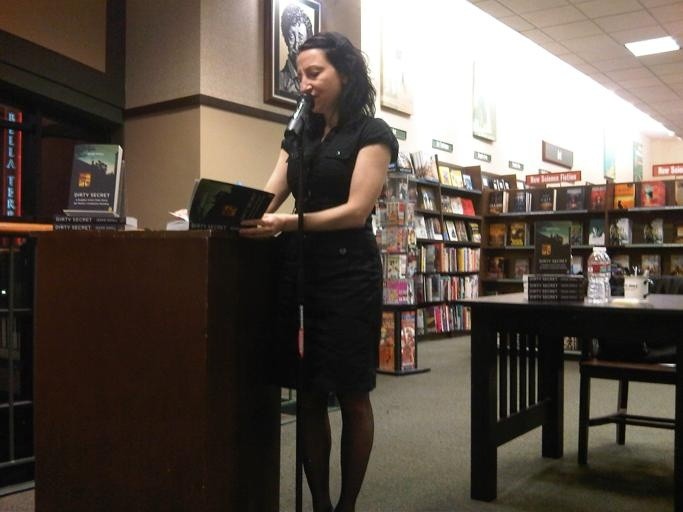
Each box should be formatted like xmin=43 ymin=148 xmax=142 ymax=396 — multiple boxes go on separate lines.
xmin=643 ymin=135 xmax=683 ymax=181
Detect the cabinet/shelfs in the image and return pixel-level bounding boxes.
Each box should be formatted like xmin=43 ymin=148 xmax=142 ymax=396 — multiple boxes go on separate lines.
xmin=379 ymin=157 xmax=485 ymax=346
xmin=484 ymin=184 xmax=668 ymax=294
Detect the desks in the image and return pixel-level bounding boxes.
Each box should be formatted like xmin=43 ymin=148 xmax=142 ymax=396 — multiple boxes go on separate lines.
xmin=448 ymin=295 xmax=683 ymax=512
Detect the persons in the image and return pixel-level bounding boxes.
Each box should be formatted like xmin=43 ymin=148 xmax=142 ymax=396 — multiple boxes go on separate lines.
xmin=276 ymin=4 xmax=316 ymax=97
xmin=238 ymin=31 xmax=399 ymax=512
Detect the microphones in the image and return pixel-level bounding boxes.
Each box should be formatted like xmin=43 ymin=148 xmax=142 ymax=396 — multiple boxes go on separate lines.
xmin=283 ymin=92 xmax=314 ymax=141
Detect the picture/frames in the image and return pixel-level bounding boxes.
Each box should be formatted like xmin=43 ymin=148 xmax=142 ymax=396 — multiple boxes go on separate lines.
xmin=263 ymin=0 xmax=326 ymax=110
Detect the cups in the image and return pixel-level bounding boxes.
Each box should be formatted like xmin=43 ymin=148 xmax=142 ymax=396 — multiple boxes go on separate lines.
xmin=624 ymin=275 xmax=654 ymax=299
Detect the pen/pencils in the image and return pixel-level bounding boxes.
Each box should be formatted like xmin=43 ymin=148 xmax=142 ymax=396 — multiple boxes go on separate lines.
xmin=625 ymin=265 xmax=649 ymax=277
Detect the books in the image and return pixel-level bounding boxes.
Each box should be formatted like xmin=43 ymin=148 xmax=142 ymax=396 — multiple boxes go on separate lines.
xmin=370 ymin=151 xmax=682 ymax=374
xmin=66 ymin=142 xmax=122 ymax=214
xmin=53 ymin=223 xmax=138 ymax=231
xmin=165 ymin=177 xmax=274 ymax=238
xmin=53 ymin=214 xmax=138 ymax=224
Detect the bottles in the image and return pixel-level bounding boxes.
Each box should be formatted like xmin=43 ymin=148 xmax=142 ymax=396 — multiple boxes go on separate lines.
xmin=588 ymin=246 xmax=612 ymax=300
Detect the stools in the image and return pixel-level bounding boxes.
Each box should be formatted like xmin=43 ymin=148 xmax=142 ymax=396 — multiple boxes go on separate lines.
xmin=577 ymin=359 xmax=683 ymax=466
